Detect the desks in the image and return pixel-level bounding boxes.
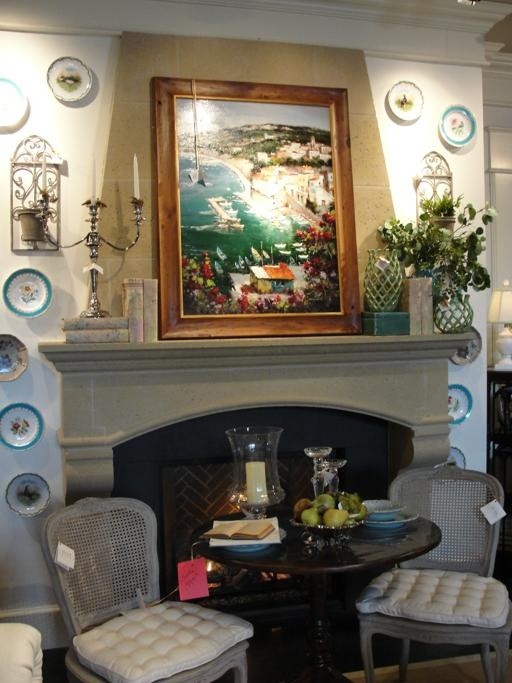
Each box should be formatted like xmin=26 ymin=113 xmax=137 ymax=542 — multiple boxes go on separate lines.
xmin=188 ymin=506 xmax=444 ymax=680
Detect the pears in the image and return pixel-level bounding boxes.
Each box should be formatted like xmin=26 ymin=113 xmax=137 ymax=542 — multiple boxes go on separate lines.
xmin=316 ymin=494 xmax=337 ymax=514
xmin=300 ymin=507 xmax=320 ymax=526
xmin=323 ymin=508 xmax=348 ymax=526
xmin=357 ymin=508 xmax=367 ymax=521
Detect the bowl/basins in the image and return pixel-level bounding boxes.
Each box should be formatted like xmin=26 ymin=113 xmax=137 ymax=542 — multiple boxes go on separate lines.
xmin=354 ymin=498 xmax=407 ymax=518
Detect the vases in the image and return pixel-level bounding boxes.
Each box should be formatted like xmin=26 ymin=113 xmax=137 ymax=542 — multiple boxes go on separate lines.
xmin=364 ymin=250 xmax=406 ymax=312
xmin=434 ymin=282 xmax=476 ymax=334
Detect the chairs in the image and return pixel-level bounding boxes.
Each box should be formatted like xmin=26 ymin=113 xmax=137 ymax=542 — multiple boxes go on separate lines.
xmin=42 ymin=495 xmax=253 ymax=683
xmin=354 ymin=468 xmax=512 ymax=681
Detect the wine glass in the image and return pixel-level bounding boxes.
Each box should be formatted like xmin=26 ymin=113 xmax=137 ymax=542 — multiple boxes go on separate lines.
xmin=225 ymin=425 xmax=288 ymax=521
xmin=304 ymin=444 xmax=347 ymax=498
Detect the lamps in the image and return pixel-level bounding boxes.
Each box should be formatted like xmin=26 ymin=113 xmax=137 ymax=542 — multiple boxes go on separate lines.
xmin=486 ymin=287 xmax=512 ymax=370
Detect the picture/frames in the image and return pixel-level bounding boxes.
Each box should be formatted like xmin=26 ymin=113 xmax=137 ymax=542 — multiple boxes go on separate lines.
xmin=150 ymin=72 xmax=364 ymax=339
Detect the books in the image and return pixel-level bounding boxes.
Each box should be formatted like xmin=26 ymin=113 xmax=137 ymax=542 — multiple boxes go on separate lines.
xmin=199 ymin=522 xmax=277 ymax=541
xmin=54 ymin=276 xmax=161 ymax=346
xmin=398 ymin=274 xmax=434 ymax=340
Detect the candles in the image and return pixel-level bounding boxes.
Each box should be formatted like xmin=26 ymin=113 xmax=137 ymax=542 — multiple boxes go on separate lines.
xmin=32 ymin=152 xmax=145 ymax=214
xmin=247 ymin=462 xmax=267 ymax=496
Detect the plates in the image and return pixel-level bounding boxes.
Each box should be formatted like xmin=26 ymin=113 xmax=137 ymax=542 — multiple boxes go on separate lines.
xmin=358 ymin=510 xmax=417 ymax=530
xmin=445 ymin=444 xmax=467 ymax=472
xmin=451 ymin=323 xmax=484 ymax=365
xmin=0 ymin=401 xmax=46 ymax=452
xmin=445 ymin=383 xmax=475 ymax=423
xmin=4 ymin=470 xmax=52 ymax=519
xmin=224 ymin=527 xmax=287 ymax=553
xmin=438 ymin=101 xmax=481 ymax=148
xmin=0 ymin=333 xmax=30 ymax=381
xmin=1 ymin=266 xmax=54 ymax=320
xmin=48 ymin=56 xmax=94 ymax=102
xmin=386 ymin=77 xmax=427 ymax=122
xmin=0 ymin=76 xmax=29 ymax=132
xmin=294 ymin=518 xmax=362 ymax=536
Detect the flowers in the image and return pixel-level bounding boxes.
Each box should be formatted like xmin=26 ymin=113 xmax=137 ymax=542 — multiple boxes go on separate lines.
xmin=381 ymin=196 xmax=500 ymax=303
